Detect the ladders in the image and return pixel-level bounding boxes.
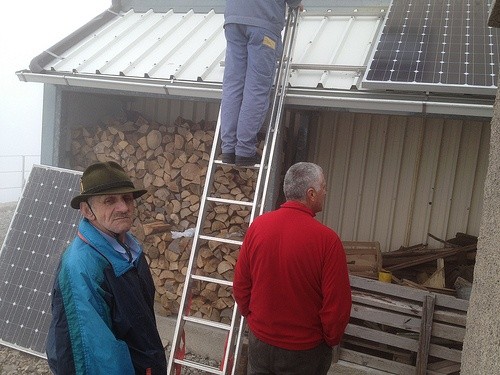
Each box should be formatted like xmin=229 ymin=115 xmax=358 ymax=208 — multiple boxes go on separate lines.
xmin=167 ymin=0 xmax=300 ymax=375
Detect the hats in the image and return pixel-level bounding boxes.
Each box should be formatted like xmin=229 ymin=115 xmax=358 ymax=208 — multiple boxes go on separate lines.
xmin=71 ymin=161 xmax=148 ymax=210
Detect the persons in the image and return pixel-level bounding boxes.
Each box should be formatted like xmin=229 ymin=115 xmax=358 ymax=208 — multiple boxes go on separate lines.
xmin=233 ymin=162 xmax=352 ymax=375
xmin=46 ymin=161 xmax=167 ymax=375
xmin=220 ymin=0 xmax=304 ymax=166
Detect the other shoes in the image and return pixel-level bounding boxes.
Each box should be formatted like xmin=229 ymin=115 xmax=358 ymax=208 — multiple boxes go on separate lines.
xmin=235 ymin=152 xmax=262 ymax=166
xmin=222 ymin=152 xmax=236 ymax=163
